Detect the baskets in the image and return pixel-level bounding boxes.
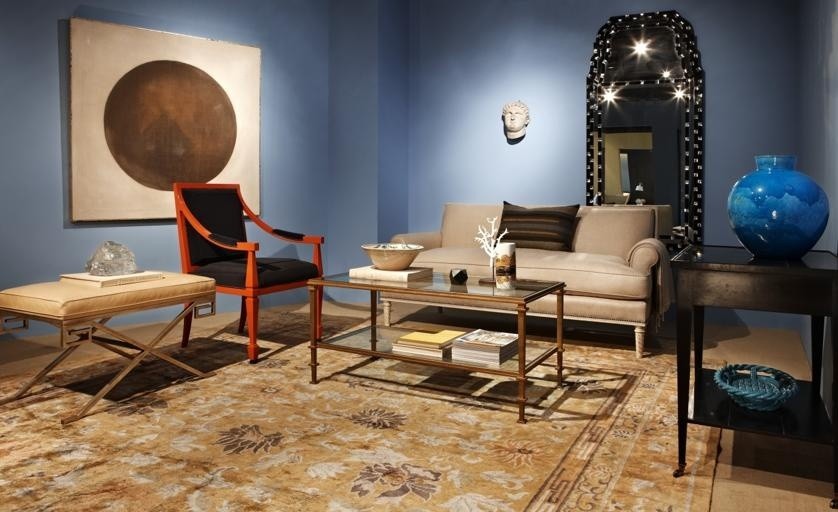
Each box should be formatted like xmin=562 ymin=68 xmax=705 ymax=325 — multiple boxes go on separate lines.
xmin=714 ymin=363 xmax=798 ymax=412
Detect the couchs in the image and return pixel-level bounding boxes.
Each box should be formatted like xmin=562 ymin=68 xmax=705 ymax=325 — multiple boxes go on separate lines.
xmin=382 ymin=204 xmax=663 ymax=359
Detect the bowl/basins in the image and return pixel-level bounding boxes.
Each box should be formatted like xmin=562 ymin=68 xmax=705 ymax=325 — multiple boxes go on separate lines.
xmin=361 ymin=243 xmax=424 ymax=271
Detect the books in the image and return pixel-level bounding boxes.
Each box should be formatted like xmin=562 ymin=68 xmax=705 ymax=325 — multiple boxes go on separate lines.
xmin=452 ymin=328 xmax=519 ymax=368
xmin=348 ymin=265 xmax=433 ymax=282
xmin=391 ymin=328 xmax=466 ymax=362
xmin=59 ymin=270 xmax=164 ymax=288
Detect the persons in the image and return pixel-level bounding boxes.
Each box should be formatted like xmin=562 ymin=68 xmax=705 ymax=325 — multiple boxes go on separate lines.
xmin=502 ymin=102 xmax=529 ymax=139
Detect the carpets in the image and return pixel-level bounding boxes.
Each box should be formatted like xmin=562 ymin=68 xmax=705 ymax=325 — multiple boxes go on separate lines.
xmin=0 ymin=311 xmax=729 ymax=512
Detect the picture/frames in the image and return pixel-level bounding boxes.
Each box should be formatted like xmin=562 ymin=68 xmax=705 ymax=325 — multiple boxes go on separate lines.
xmin=68 ymin=17 xmax=261 ymax=222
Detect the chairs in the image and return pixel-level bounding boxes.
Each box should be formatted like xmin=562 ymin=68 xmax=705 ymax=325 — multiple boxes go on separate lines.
xmin=173 ymin=181 xmax=326 ymax=364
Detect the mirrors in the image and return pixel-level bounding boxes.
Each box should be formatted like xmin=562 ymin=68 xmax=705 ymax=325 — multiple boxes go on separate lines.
xmin=584 ymin=12 xmax=705 ymax=245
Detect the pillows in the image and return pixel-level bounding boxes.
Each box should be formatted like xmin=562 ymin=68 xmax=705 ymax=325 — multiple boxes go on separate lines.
xmin=494 ymin=201 xmax=581 ymax=252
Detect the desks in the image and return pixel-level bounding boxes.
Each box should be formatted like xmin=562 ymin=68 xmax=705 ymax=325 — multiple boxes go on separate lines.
xmin=0 ymin=270 xmax=214 ymax=424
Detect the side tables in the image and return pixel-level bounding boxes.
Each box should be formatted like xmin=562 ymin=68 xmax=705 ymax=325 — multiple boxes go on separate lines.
xmin=671 ymin=244 xmax=838 ymax=509
